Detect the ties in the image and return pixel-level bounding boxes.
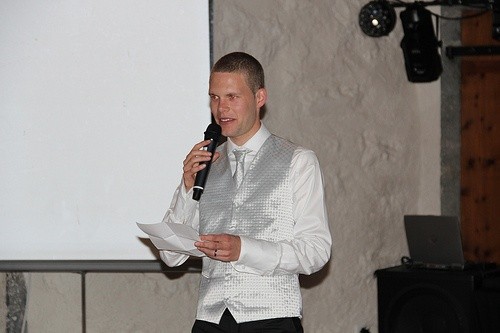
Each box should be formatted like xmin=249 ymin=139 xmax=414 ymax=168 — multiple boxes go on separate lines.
xmin=232 ymin=147 xmax=254 ymax=190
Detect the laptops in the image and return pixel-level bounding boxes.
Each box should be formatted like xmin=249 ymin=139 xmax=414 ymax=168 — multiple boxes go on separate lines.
xmin=404 ymin=215 xmax=496 ymax=271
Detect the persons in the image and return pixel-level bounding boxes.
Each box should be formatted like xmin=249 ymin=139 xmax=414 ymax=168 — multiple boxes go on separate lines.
xmin=157 ymin=52 xmax=332 ymax=333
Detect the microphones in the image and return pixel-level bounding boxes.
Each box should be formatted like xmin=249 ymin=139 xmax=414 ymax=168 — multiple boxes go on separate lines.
xmin=193 ymin=123 xmax=221 ymax=200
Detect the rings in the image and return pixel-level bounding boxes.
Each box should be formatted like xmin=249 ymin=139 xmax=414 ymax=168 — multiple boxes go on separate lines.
xmin=214 ymin=249 xmax=217 ymax=256
